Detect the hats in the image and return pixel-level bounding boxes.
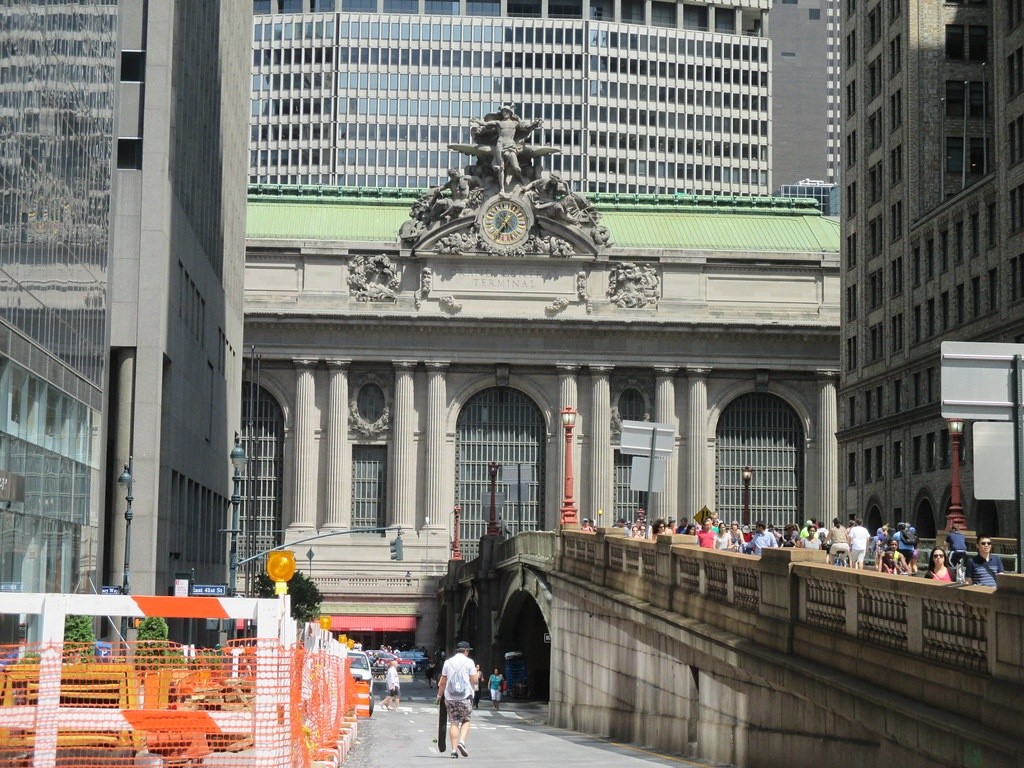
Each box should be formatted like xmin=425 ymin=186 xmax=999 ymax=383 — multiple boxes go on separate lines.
xmin=833 ymin=517 xmax=841 ymax=524
xmin=742 ymin=526 xmax=750 ymax=534
xmin=806 ymin=520 xmax=813 ymax=526
xmin=909 ymin=527 xmax=915 ymax=532
xmin=583 ymin=518 xmax=588 ymax=522
xmin=877 ymin=528 xmax=883 ymax=535
xmin=456 ymin=641 xmax=473 ymax=651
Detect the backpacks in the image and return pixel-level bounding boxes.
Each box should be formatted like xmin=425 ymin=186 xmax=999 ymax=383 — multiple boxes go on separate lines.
xmin=900 ymin=529 xmax=919 ymax=544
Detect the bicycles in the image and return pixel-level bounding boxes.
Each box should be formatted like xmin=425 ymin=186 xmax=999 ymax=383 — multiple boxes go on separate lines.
xmin=824 ymin=545 xmax=847 ymax=567
xmin=955 ymin=559 xmax=965 ymax=584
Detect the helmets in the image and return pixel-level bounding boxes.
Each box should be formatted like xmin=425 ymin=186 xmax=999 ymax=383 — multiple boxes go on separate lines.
xmin=731 ymin=521 xmax=738 ymax=528
xmin=897 ymin=522 xmax=904 ymax=530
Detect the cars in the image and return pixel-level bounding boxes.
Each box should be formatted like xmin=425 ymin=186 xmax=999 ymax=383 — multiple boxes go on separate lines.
xmin=364 ymin=650 xmax=431 ymax=675
xmin=347 ymin=651 xmax=373 ymax=695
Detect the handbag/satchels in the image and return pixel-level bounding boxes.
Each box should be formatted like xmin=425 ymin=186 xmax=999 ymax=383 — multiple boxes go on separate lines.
xmin=501 ymin=674 xmax=507 ymax=691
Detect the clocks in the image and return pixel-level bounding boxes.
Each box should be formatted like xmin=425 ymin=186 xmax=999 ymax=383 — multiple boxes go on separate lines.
xmin=481 ymin=199 xmax=527 ymax=244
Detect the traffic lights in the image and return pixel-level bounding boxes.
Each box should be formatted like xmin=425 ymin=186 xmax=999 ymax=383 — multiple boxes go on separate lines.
xmin=390 ymin=539 xmax=403 ymax=560
xmin=128 ymin=617 xmax=146 ymax=630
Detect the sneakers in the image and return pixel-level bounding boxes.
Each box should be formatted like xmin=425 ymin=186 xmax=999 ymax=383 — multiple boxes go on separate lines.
xmin=458 ymin=742 xmax=468 ymax=757
xmin=451 ymin=751 xmax=458 ymax=758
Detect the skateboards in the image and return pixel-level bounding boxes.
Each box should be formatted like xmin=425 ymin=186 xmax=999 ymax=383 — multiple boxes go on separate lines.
xmin=432 ymin=693 xmax=447 ymax=752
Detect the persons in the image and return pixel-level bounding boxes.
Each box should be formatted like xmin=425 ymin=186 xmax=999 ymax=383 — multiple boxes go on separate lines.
xmin=581 ymin=516 xmax=597 ymax=532
xmin=433 ymin=168 xmax=482 ymax=201
xmin=613 ymin=508 xmax=871 ymax=570
xmin=437 ymin=641 xmax=477 ymax=758
xmin=427 ymin=659 xmax=436 ymax=689
xmin=521 ymin=172 xmax=570 ymax=199
xmin=871 ymin=521 xmax=918 ymax=576
xmin=925 ymin=523 xmax=1004 ymax=589
xmin=379 ymin=644 xmax=427 ymax=656
xmin=471 ymin=106 xmax=543 ymax=196
xmin=472 ymin=665 xmax=485 ymax=710
xmin=379 ymin=661 xmax=401 ymax=712
xmin=488 ymin=668 xmax=504 ymax=711
xmin=365 ymin=650 xmax=386 ymax=678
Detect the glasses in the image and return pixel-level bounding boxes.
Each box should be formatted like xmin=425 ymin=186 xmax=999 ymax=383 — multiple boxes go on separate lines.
xmin=659 ymin=526 xmax=665 ymax=528
xmin=980 ymin=542 xmax=992 ymax=545
xmin=933 ymin=554 xmax=943 ymax=558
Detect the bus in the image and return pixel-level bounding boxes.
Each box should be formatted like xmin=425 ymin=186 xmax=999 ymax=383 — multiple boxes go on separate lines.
xmin=225 ymin=638 xmax=256 ymax=656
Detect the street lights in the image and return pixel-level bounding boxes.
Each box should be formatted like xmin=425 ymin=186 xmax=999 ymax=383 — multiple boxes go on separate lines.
xmin=453 ymin=505 xmax=462 ymax=560
xmin=487 ymin=462 xmax=499 ymax=536
xmin=742 ymin=467 xmax=752 ymax=525
xmin=118 ymin=456 xmax=133 ymax=663
xmin=944 ymin=419 xmax=967 ymax=530
xmin=560 ymin=406 xmax=578 ymax=525
xmin=219 ymin=440 xmax=248 ymax=646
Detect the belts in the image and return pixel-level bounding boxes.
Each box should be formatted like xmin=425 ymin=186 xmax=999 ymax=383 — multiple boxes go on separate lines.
xmin=954 ymin=550 xmax=966 ymax=552
